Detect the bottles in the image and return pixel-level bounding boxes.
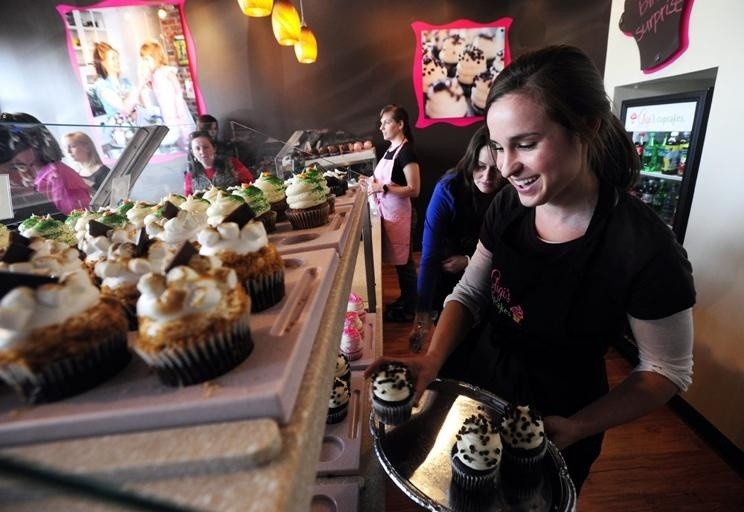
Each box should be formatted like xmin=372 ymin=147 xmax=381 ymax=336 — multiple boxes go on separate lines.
xmin=632 ymin=131 xmax=691 ymax=224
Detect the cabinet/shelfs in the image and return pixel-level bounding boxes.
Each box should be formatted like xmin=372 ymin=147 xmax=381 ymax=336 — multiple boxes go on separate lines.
xmin=0 ymin=181 xmax=382 ymax=512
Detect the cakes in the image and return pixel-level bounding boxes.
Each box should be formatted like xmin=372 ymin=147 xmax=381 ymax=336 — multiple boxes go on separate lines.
xmin=285 ymin=165 xmax=350 ymax=230
xmin=368 ymin=359 xmax=417 ymax=428
xmin=418 ymin=26 xmax=506 ymax=120
xmin=335 ymin=352 xmax=352 ymax=387
xmin=494 ymin=401 xmax=550 ymax=469
xmin=448 ymin=413 xmax=505 ymax=497
xmin=325 ymin=376 xmax=350 ymax=427
xmin=342 ymin=309 xmax=368 ymax=339
xmin=347 ymin=292 xmax=367 ymax=323
xmin=339 ymin=324 xmax=365 ymax=363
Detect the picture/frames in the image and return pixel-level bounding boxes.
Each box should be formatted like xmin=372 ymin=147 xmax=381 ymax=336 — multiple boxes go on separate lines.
xmin=411 ymin=17 xmax=513 ymax=129
xmin=56 ymin=0 xmax=208 ymax=168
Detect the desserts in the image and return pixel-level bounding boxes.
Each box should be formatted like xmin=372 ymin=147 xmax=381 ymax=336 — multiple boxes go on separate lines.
xmin=0 ymin=163 xmax=286 ymax=414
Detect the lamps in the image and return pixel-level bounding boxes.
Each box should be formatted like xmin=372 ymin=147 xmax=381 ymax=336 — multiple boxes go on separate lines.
xmin=271 ymin=0 xmax=303 ymax=45
xmin=294 ymin=0 xmax=318 ymax=64
xmin=238 ymin=0 xmax=274 ymax=17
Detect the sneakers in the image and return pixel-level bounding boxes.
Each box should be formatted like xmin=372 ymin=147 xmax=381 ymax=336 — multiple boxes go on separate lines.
xmin=383 ymin=305 xmax=415 ymax=322
xmin=385 ymin=295 xmax=415 ymax=311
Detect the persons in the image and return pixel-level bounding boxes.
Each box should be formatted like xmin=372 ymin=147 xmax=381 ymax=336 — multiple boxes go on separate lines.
xmin=65 ymin=131 xmax=110 ymax=194
xmin=124 ymin=40 xmax=196 ymax=148
xmin=363 ymin=44 xmax=697 ymax=500
xmin=87 ymin=41 xmax=130 ymax=130
xmin=0 ymin=111 xmax=66 ymax=224
xmin=15 ymin=113 xmax=92 ymax=216
xmin=407 ymin=124 xmax=511 ymax=355
xmin=186 ymin=114 xmax=233 ymax=163
xmin=184 ymin=131 xmax=255 ymax=196
xmin=366 ymin=104 xmax=421 ymax=324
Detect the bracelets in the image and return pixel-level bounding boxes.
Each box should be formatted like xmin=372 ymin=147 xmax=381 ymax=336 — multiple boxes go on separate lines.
xmin=463 ymin=254 xmax=472 ymax=271
xmin=382 ymin=184 xmax=389 ymax=197
